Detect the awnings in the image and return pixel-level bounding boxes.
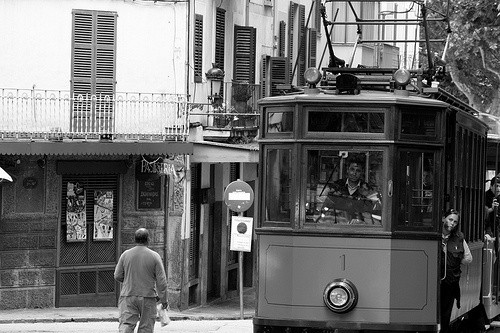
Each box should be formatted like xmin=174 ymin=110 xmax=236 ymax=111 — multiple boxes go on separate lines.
xmin=0 ymin=139 xmax=193 ymax=157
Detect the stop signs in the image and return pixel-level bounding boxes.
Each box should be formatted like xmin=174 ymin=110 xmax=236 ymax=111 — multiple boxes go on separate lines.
xmin=223 ymin=181 xmax=254 ymax=212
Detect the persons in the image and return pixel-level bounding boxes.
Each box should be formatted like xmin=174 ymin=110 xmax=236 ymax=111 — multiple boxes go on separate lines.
xmin=326 ymin=156 xmax=382 ymax=224
xmin=484 ymin=176 xmax=500 ymax=300
xmin=113 ymin=227 xmax=169 ymax=333
xmin=437 ymin=208 xmax=473 ymax=333
xmin=423 ymin=168 xmax=433 ymax=212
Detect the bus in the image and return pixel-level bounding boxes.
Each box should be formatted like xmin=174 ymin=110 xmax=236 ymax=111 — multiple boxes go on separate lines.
xmin=252 ymin=57 xmax=500 ymax=333
